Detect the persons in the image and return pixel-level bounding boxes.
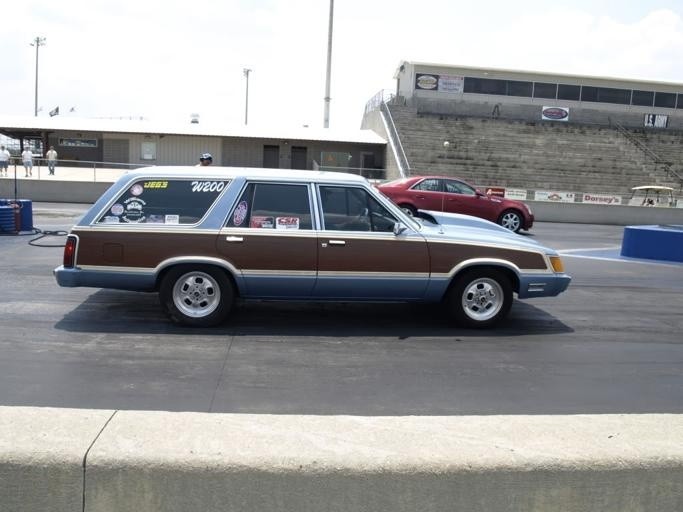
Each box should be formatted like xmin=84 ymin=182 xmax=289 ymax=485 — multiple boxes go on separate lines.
xmin=196 ymin=153 xmax=212 ymax=166
xmin=0 ymin=146 xmax=10 ymax=177
xmin=46 ymin=146 xmax=58 ymax=175
xmin=21 ymin=147 xmax=32 ymax=177
xmin=320 ymin=187 xmax=353 ymax=230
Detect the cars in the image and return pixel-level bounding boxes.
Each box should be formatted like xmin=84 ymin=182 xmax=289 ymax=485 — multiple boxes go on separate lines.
xmin=373 ymin=176 xmax=535 ymax=234
xmin=52 ymin=167 xmax=573 ymax=331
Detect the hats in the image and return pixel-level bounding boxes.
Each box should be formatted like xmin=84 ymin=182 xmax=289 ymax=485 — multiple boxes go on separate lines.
xmin=200 ymin=153 xmax=212 ymax=160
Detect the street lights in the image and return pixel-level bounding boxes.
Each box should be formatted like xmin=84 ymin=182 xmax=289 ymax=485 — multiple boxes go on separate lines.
xmin=443 ymin=140 xmax=449 ymax=180
xmin=30 ymin=37 xmax=46 ymax=116
xmin=243 ymin=68 xmax=252 ymax=125
